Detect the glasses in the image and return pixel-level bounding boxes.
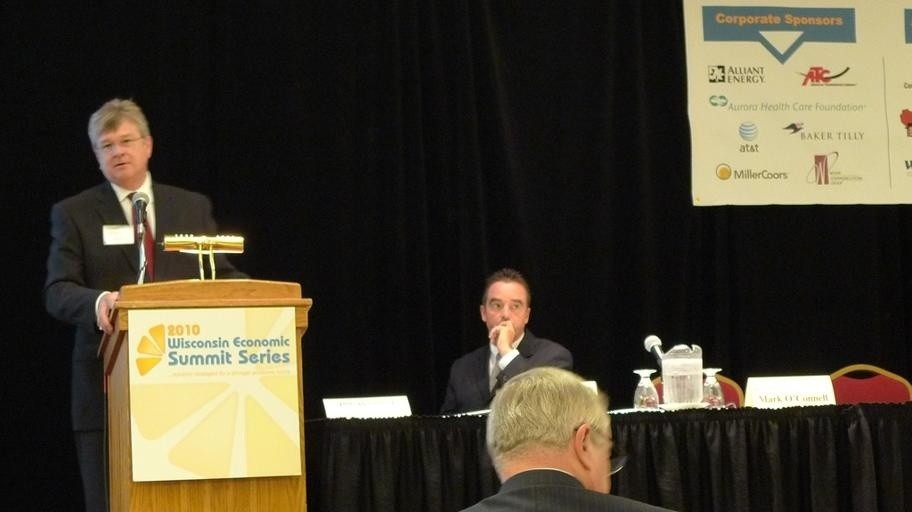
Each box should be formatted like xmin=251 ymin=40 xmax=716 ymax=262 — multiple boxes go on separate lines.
xmin=576 ymin=421 xmax=629 ymax=478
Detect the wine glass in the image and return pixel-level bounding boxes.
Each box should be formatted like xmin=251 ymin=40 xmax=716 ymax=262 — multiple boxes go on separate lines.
xmin=701 ymin=368 xmax=726 ymax=406
xmin=632 ymin=368 xmax=660 ymax=409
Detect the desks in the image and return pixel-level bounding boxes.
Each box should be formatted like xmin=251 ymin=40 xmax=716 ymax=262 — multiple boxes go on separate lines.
xmin=308 ymin=400 xmax=912 ymax=510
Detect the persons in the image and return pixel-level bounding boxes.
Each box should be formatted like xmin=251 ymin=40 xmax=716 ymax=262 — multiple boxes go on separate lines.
xmin=42 ymin=96 xmax=251 ymax=512
xmin=454 ymin=365 xmax=674 ymax=512
xmin=438 ymin=268 xmax=576 ymax=419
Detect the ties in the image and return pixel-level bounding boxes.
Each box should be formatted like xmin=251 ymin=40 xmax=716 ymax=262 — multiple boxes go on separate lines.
xmin=488 ymin=352 xmax=502 ymax=395
xmin=126 ymin=190 xmax=158 ymax=283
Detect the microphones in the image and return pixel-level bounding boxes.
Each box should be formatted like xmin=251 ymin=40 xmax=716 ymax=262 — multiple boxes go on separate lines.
xmin=132 ymin=191 xmax=150 ymax=240
xmin=644 ymin=335 xmax=666 ymax=365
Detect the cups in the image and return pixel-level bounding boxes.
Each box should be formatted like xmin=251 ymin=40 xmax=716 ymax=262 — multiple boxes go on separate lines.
xmin=662 ymin=344 xmax=704 ymax=405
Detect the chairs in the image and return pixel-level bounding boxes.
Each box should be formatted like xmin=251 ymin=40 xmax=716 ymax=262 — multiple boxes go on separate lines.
xmin=640 ymin=373 xmax=744 ymax=408
xmin=830 ymin=364 xmax=911 ymax=406
